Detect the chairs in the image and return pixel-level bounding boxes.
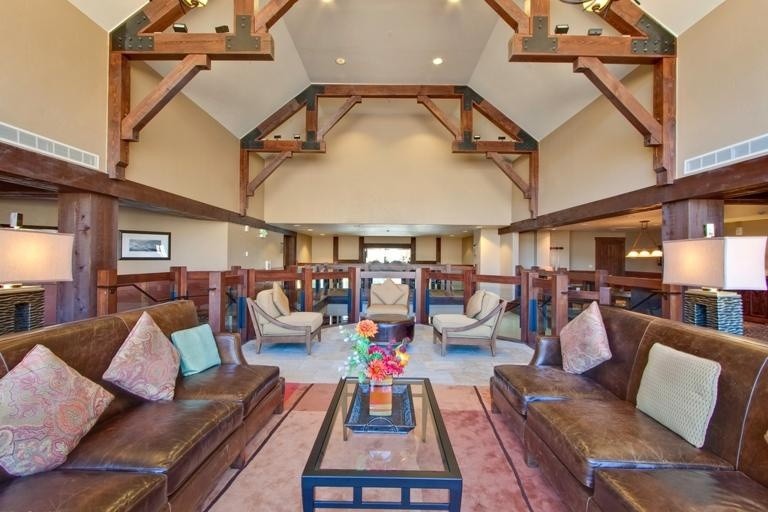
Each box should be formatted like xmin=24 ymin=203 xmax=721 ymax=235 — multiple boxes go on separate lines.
xmin=366 ymin=278 xmax=410 ymax=322
xmin=247 ymin=282 xmax=324 ymax=355
xmin=433 ymin=290 xmax=507 ymax=357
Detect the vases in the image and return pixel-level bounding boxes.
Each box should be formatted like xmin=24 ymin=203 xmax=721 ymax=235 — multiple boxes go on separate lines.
xmin=368 ymin=377 xmax=393 ymax=416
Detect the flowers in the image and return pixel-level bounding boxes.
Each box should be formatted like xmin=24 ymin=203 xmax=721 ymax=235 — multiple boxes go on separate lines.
xmin=339 ymin=319 xmax=411 ymax=377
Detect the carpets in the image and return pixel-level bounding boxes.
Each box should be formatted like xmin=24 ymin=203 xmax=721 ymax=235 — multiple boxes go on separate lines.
xmin=203 ymin=382 xmax=570 ymax=512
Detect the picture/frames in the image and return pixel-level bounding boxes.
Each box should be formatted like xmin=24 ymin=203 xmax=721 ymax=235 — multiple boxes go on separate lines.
xmin=117 ymin=230 xmax=172 ymax=260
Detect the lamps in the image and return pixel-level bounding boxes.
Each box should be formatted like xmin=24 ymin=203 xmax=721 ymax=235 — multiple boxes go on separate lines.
xmin=625 ymin=221 xmax=662 ymax=258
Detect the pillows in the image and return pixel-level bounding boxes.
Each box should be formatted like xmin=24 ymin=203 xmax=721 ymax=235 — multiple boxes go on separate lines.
xmin=170 ymin=324 xmax=222 ymax=376
xmin=559 ymin=300 xmax=611 ymax=373
xmin=103 ymin=311 xmax=181 ymax=401
xmin=273 ymin=283 xmax=290 ymax=315
xmin=465 ymin=289 xmax=485 ymax=317
xmin=0 ymin=343 xmax=115 ymax=477
xmin=636 ymin=342 xmax=721 ymax=447
xmin=374 ymin=279 xmax=404 ymax=305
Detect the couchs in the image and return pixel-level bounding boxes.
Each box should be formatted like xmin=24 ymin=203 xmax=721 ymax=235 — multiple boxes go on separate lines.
xmin=0 ymin=300 xmax=285 ymax=511
xmin=489 ymin=304 xmax=768 ymax=512
